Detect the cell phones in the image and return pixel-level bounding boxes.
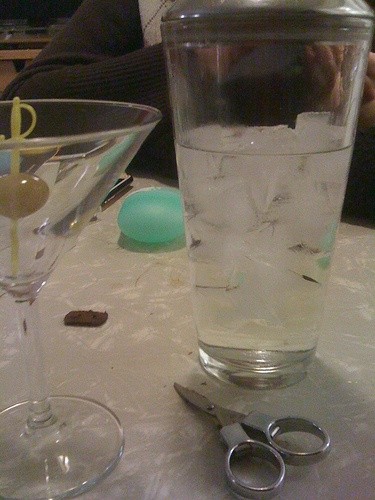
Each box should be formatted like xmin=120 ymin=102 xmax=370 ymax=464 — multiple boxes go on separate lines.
xmin=102 ymin=174 xmax=133 ymax=206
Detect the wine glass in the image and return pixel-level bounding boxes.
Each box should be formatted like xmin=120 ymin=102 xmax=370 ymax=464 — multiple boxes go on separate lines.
xmin=0 ymin=99 xmax=163 ymax=500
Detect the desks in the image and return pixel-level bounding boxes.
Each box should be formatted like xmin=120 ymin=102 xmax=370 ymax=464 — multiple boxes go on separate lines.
xmin=0 ymin=132 xmax=374 ymax=500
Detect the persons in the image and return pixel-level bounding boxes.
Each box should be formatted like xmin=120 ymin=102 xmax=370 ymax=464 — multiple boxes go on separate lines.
xmin=0 ymin=0 xmax=375 ymax=219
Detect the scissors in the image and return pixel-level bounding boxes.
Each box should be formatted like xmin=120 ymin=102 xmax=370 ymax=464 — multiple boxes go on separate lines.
xmin=174 ymin=383 xmax=331 ymax=500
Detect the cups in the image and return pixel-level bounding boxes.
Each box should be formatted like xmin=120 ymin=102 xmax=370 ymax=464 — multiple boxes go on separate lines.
xmin=159 ymin=0 xmax=374 ymax=390
